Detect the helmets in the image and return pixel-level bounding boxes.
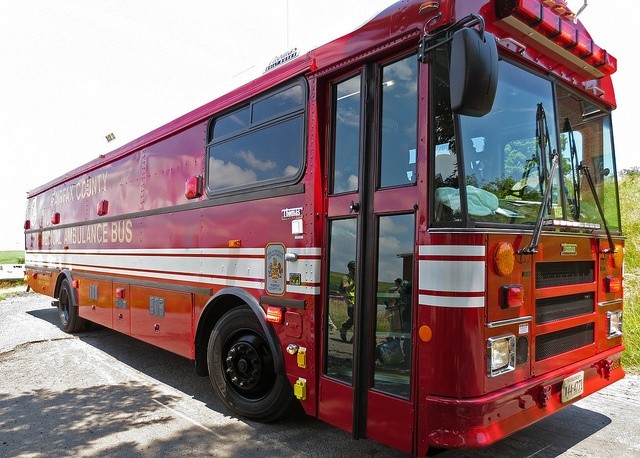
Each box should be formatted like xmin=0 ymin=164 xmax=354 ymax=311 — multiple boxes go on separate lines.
xmin=347 ymin=261 xmax=355 ymax=269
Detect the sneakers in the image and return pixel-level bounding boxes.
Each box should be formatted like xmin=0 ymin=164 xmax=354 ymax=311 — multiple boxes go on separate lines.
xmin=339 ymin=327 xmax=347 ymax=341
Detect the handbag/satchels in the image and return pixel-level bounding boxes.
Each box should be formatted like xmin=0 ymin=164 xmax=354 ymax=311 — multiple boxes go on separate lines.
xmin=434 ymin=185 xmax=498 ymax=222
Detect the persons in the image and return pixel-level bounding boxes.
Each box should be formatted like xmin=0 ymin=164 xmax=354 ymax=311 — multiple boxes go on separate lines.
xmin=393 ymin=277 xmax=410 ymax=330
xmin=339 ymin=260 xmax=356 ymax=344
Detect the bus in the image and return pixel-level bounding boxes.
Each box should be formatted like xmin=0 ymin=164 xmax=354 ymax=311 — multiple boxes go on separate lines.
xmin=23 ymin=0 xmax=629 ymax=458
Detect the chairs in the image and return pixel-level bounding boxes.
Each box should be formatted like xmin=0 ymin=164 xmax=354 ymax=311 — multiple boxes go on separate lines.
xmin=414 ymin=154 xmax=458 ymax=182
